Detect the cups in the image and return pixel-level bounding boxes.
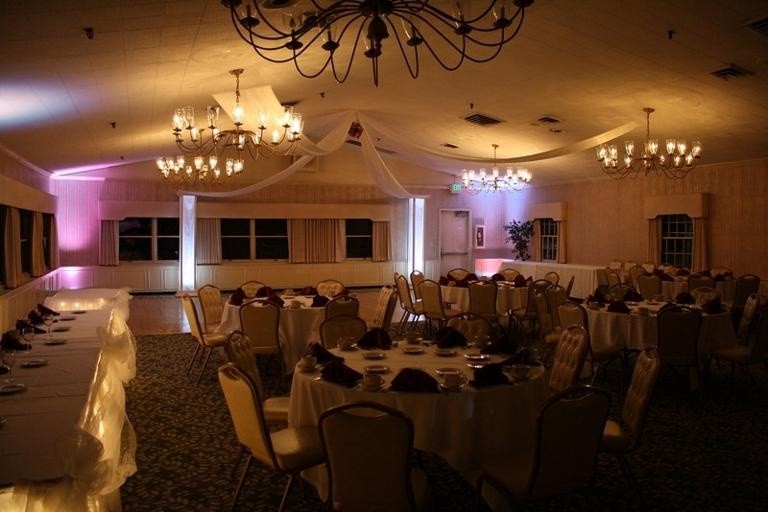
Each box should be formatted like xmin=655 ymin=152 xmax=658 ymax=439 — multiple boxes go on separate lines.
xmin=366 ymin=373 xmax=384 ymax=388
xmin=301 ymin=331 xmax=419 ymax=367
xmin=511 ymin=364 xmax=531 ymax=376
xmin=441 ymin=374 xmax=467 ymax=387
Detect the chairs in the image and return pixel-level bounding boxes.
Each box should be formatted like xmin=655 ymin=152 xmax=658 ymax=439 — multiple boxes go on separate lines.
xmin=606 ymin=260 xmax=760 ymax=389
xmin=181 ymin=267 xmax=663 ymax=512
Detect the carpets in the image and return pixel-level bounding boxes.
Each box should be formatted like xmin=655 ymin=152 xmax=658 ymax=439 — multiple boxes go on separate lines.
xmin=116 ymin=320 xmax=768 ymax=512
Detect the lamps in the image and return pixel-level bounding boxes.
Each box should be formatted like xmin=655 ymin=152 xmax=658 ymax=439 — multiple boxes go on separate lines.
xmin=460 ymin=144 xmax=533 ymax=197
xmin=154 ymin=151 xmax=245 ymax=189
xmin=595 ymin=108 xmax=703 ymax=180
xmin=217 ymin=0 xmax=537 ymax=89
xmin=170 ymin=68 xmax=304 ymax=159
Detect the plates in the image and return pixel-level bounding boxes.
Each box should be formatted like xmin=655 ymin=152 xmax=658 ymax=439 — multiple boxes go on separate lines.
xmin=282 ymin=296 xmax=314 ymax=299
xmin=469 ymin=279 xmax=663 ymax=317
xmin=0 ymin=309 xmax=87 ymax=395
xmin=363 ymin=345 xmax=489 ymax=375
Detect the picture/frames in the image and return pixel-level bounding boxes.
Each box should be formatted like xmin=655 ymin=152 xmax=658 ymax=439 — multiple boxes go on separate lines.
xmin=474 ymin=225 xmax=485 ymax=249
